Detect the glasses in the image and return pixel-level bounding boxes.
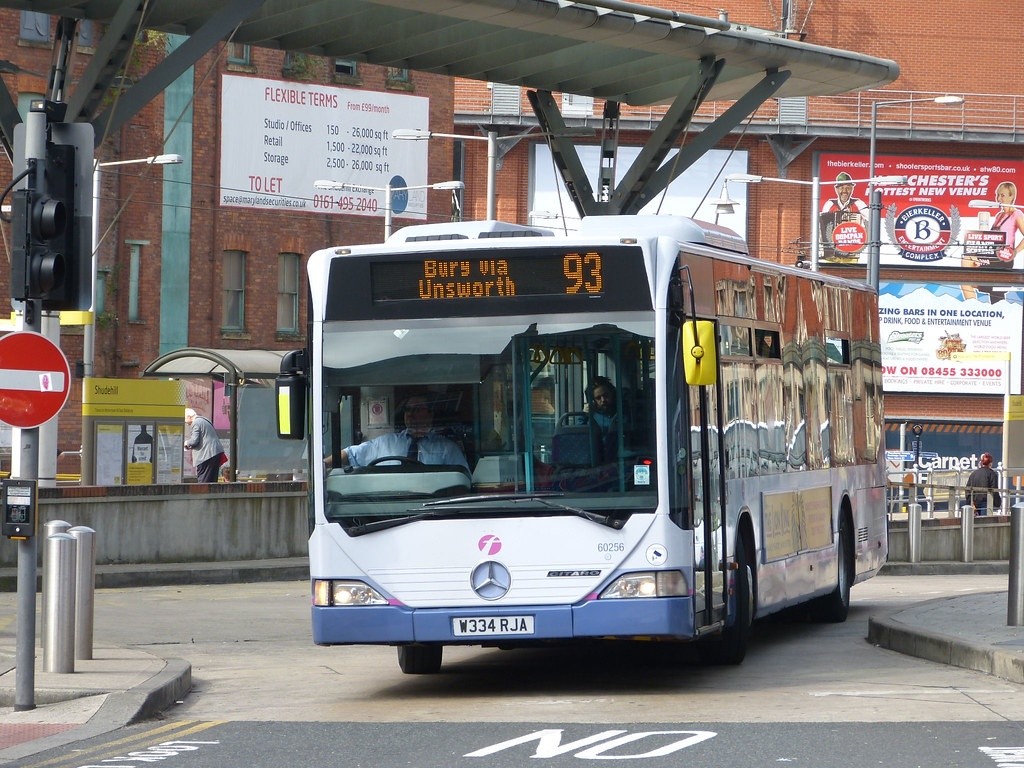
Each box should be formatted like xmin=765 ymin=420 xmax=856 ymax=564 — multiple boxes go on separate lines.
xmin=401 ymin=405 xmax=431 ymax=413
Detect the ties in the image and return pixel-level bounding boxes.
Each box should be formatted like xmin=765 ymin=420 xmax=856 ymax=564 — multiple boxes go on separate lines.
xmin=406 ymin=434 xmax=423 ymax=466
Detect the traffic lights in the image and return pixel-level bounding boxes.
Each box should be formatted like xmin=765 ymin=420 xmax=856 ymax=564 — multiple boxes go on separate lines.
xmin=9 ymin=189 xmax=70 ymax=298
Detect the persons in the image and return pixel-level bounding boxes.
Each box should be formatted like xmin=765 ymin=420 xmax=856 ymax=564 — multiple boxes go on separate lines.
xmin=183 ymin=408 xmax=227 ymax=483
xmin=577 ymin=376 xmax=647 ymax=432
xmin=820 ymin=168 xmax=869 ymax=264
xmin=988 ymin=180 xmax=1024 ymax=268
xmin=965 ymin=453 xmax=1002 ymax=516
xmin=322 ymin=392 xmax=474 ymax=477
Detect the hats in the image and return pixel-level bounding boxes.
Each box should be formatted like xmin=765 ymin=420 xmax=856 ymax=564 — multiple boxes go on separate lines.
xmin=836 ymin=174 xmax=856 ymax=189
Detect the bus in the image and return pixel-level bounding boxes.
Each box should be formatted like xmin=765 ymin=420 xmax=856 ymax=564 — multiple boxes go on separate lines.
xmin=274 ymin=215 xmax=890 ymax=675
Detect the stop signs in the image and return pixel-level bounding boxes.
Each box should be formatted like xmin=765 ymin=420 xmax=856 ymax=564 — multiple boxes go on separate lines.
xmin=0 ymin=330 xmax=72 ymax=431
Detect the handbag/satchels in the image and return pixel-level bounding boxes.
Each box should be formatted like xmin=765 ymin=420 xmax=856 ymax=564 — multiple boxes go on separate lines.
xmin=218 ymin=452 xmax=228 ymax=467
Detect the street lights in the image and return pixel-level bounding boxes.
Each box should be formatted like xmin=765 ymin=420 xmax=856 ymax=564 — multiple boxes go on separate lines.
xmin=310 ymin=179 xmax=467 ymax=244
xmin=867 ymin=96 xmax=966 ymax=284
xmin=708 ymin=173 xmax=910 ymax=277
xmin=388 ymin=127 xmax=598 ymax=220
xmin=79 ymin=154 xmax=185 ymax=489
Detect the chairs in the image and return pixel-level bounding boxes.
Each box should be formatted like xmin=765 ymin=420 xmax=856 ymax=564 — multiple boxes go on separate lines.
xmin=551 ymin=410 xmax=640 ymax=471
